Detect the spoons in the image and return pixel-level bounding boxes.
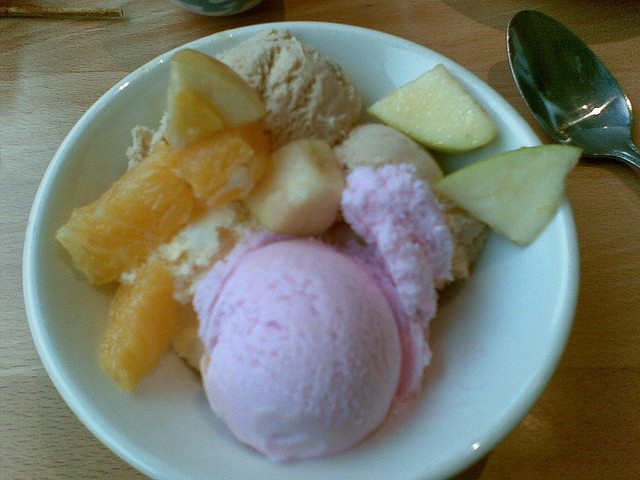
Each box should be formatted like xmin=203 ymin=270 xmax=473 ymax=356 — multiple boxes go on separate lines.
xmin=507 ymin=9 xmax=640 ymax=173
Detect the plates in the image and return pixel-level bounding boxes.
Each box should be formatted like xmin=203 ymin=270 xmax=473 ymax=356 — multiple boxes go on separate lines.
xmin=20 ymin=19 xmax=583 ymax=480
